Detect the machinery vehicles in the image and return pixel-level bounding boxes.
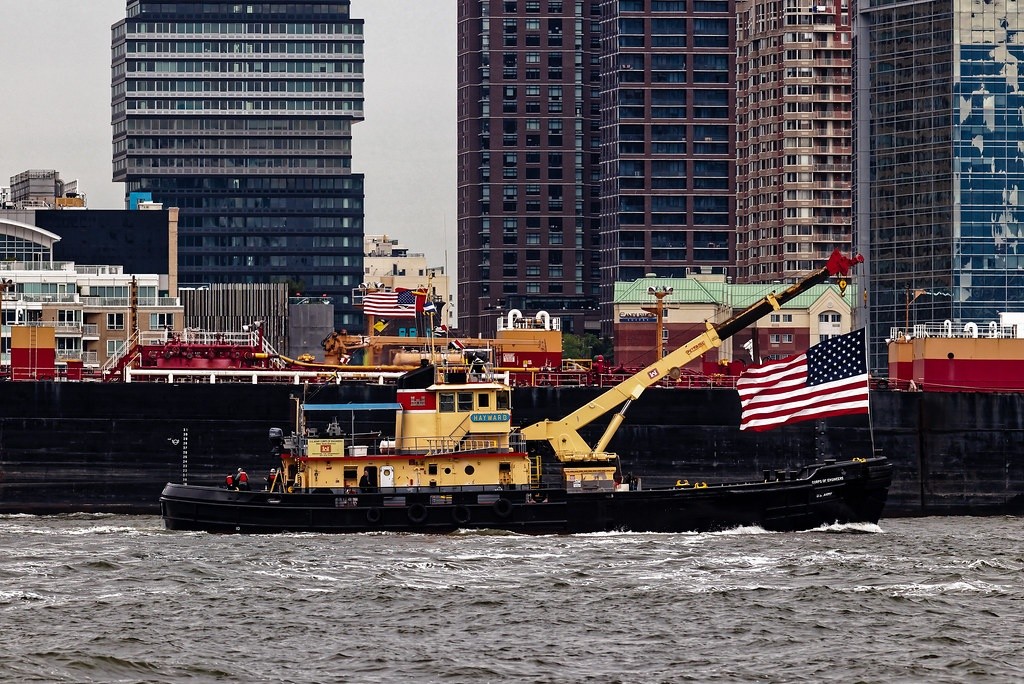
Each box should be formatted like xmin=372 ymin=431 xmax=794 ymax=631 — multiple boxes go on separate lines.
xmin=523 ymin=252 xmax=866 ymax=506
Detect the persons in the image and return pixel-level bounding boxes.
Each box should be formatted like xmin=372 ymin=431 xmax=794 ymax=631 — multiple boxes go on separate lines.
xmin=359 ymin=471 xmax=370 ymax=492
xmin=225 ymin=468 xmax=249 ymax=491
xmin=264 ymin=469 xmax=279 ymax=492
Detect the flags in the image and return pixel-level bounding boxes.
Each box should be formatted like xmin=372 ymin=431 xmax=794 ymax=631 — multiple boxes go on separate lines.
xmin=737 ymin=327 xmax=871 ymax=433
xmin=423 ymin=302 xmax=437 ymax=315
xmin=449 ymin=340 xmax=466 ymax=350
xmin=363 ymin=291 xmax=418 ymax=317
xmin=434 ymin=325 xmax=449 ymax=336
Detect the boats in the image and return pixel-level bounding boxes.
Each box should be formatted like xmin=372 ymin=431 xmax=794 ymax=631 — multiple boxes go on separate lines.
xmin=156 ymin=277 xmax=896 ymax=534
